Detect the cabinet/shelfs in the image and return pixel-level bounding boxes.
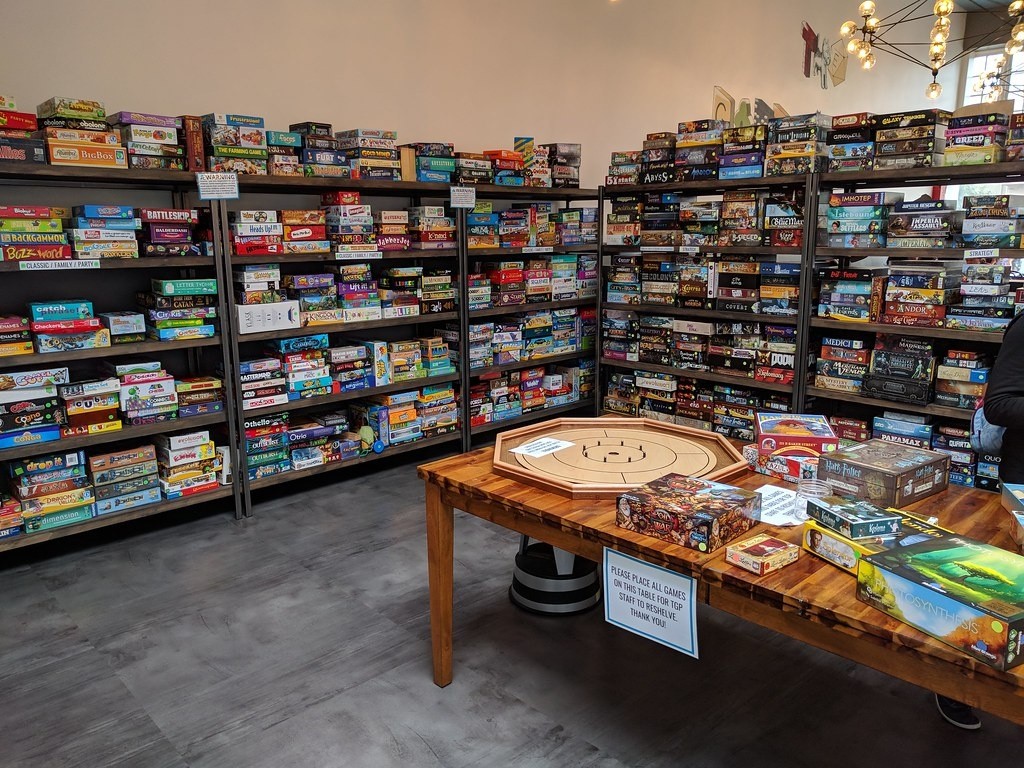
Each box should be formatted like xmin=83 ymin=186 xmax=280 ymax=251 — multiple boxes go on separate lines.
xmin=0 ymin=158 xmax=242 ymax=551
xmin=183 ymin=174 xmax=468 ymax=517
xmin=595 ymin=172 xmax=812 ymax=414
xmin=416 ymin=182 xmax=618 ymax=452
xmin=798 ymin=162 xmax=1023 ymax=413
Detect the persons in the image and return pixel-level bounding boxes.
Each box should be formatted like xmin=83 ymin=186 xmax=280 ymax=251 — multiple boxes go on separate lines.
xmin=934 ymin=305 xmax=1024 ymax=730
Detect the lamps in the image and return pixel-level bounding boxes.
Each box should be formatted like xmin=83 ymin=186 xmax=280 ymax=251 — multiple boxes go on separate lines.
xmin=840 ymin=0 xmax=1024 ymax=99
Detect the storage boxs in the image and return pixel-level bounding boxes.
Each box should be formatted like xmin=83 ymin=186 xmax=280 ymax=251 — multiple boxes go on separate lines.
xmin=0 ymin=92 xmax=611 ymax=537
xmin=602 ymin=99 xmax=1024 ymax=672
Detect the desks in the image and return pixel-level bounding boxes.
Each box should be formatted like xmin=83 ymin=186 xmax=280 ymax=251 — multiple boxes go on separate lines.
xmin=417 ymin=414 xmax=1024 ymax=727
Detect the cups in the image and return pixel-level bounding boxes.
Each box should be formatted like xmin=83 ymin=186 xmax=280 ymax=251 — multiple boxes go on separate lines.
xmin=794 ymin=479 xmax=834 ymax=521
